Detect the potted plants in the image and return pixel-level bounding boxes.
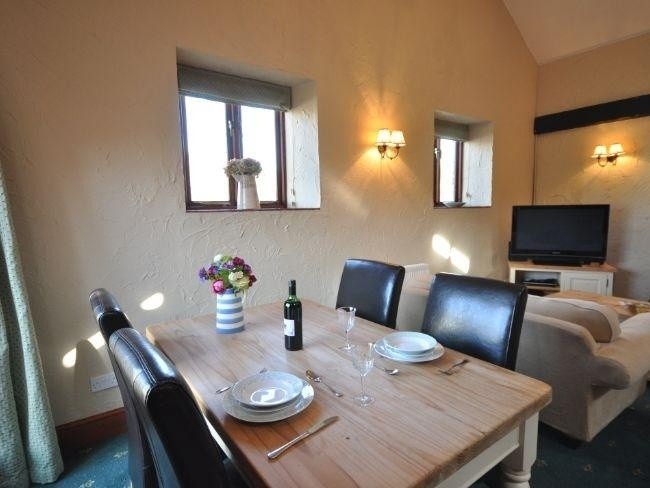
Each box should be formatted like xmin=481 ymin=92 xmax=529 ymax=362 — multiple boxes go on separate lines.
xmin=224 ymin=155 xmax=266 ymax=210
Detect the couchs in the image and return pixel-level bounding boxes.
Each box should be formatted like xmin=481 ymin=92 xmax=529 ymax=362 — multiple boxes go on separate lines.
xmin=396 ymin=263 xmax=650 ymax=444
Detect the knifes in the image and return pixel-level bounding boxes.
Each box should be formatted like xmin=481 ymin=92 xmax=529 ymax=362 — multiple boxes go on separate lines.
xmin=266 ymin=418 xmax=340 ymax=459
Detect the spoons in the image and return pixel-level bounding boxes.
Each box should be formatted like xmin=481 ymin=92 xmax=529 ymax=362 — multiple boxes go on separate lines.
xmin=307 ymin=367 xmax=341 ymax=397
xmin=375 ymin=365 xmax=399 ymax=375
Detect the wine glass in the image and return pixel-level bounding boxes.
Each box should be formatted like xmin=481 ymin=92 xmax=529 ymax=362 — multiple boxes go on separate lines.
xmin=350 ymin=342 xmax=376 ymax=406
xmin=336 ymin=305 xmax=356 ymax=353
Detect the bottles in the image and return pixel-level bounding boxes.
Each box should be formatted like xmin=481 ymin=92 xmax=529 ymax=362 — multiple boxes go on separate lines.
xmin=215 ymin=288 xmax=246 ymax=334
xmin=283 ymin=280 xmax=302 ymax=351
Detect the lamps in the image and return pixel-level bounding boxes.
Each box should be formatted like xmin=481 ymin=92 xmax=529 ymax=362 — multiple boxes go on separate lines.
xmin=590 ymin=140 xmax=624 ymax=168
xmin=375 ymin=126 xmax=406 ymax=158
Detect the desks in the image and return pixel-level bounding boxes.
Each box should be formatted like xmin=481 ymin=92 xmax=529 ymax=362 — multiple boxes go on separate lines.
xmin=544 ymin=289 xmax=650 ymax=323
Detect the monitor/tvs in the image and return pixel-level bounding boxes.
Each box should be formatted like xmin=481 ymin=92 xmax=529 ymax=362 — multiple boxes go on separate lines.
xmin=509 ymin=204 xmax=611 ymax=265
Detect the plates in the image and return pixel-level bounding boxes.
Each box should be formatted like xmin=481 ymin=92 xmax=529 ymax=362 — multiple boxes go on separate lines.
xmin=374 ymin=339 xmax=445 ymax=363
xmin=443 ymin=202 xmax=465 ymax=207
xmin=223 ymin=379 xmax=315 ymax=423
xmin=383 ymin=332 xmax=437 ymax=355
xmin=232 ymin=371 xmax=303 ymax=407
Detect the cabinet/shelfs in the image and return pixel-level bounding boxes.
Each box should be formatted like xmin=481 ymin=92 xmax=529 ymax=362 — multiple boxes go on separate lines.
xmin=507 ymin=259 xmax=616 ymax=298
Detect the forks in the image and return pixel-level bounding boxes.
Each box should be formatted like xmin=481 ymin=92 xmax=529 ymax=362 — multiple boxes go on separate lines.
xmin=438 ymin=359 xmax=469 ymax=375
